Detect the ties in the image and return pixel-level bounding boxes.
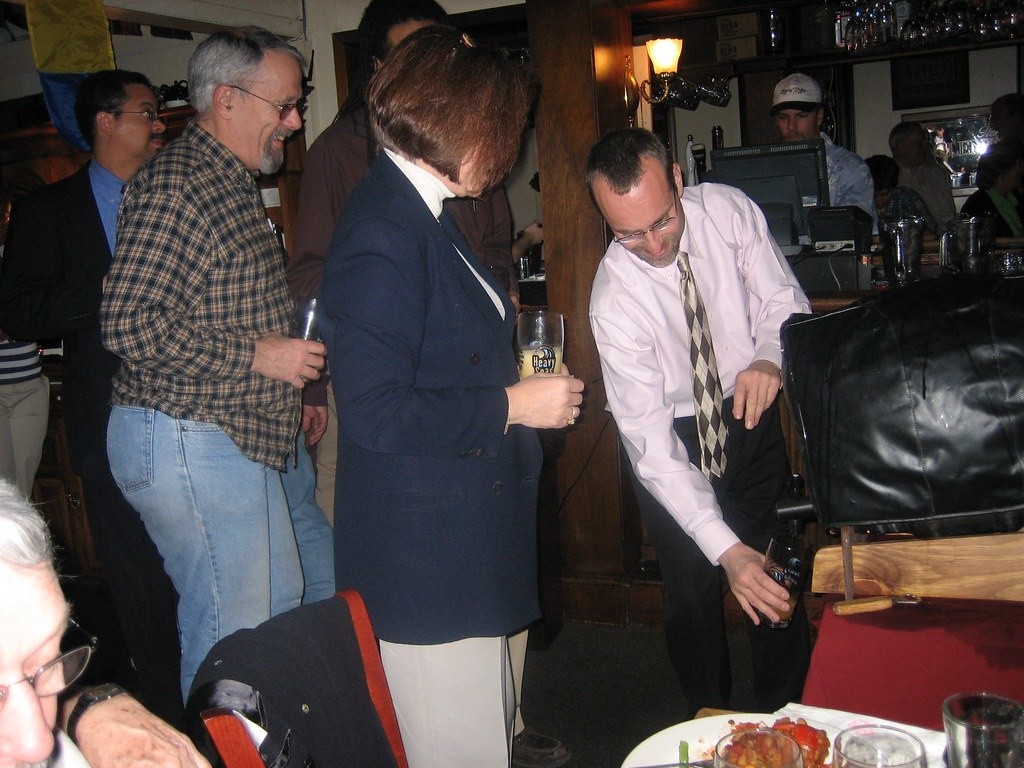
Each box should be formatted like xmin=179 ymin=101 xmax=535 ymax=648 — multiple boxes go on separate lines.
xmin=677 ymin=251 xmax=730 ymax=489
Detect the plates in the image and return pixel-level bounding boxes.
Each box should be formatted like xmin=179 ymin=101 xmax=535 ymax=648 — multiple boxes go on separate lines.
xmin=621 ymin=713 xmax=842 ymax=768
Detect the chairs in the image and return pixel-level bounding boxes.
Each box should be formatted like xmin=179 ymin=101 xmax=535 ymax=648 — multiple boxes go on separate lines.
xmin=197 ymin=589 xmax=408 ymax=768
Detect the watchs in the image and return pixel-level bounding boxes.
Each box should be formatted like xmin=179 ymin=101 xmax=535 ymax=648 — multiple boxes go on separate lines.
xmin=67 ymin=682 xmax=128 ymax=744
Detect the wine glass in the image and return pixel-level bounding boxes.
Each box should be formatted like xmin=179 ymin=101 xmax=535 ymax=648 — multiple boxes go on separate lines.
xmin=899 ymin=0 xmax=1024 ymax=40
xmin=846 ymin=0 xmax=897 ymax=51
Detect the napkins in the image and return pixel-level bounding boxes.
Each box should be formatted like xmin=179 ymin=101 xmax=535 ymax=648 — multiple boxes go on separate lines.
xmin=773 ymin=702 xmax=949 ymax=768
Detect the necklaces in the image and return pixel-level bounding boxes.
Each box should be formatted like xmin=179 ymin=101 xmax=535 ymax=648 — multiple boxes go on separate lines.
xmin=887 ymin=121 xmax=957 ymax=229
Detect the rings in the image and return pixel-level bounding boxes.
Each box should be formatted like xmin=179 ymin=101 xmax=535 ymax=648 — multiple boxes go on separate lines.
xmin=567 ymin=409 xmax=575 ymax=424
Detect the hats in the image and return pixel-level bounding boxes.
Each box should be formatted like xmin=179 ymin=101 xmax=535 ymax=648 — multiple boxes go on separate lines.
xmin=770 ymin=73 xmax=822 ymax=116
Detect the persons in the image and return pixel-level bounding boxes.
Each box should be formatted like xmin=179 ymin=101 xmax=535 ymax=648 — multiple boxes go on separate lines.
xmin=0 ymin=0 xmax=546 ymax=703
xmin=863 ymin=154 xmax=939 ymax=243
xmin=0 ymin=476 xmax=212 ymax=768
xmin=584 ymin=126 xmax=814 ymax=720
xmin=769 ymin=73 xmax=873 ymax=219
xmin=319 ymin=23 xmax=583 ymax=768
xmin=960 ymin=93 xmax=1024 ymax=237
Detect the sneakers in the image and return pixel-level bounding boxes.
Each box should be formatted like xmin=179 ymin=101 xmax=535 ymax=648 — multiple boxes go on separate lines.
xmin=512 ymin=729 xmax=574 ymax=768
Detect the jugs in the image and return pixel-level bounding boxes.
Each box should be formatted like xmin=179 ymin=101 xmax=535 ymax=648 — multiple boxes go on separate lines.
xmin=878 ymin=214 xmax=925 ymax=285
xmin=939 ymin=215 xmax=994 ymax=277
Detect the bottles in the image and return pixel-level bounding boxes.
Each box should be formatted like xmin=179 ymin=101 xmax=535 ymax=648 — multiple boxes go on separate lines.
xmin=835 ymin=0 xmax=854 ymax=48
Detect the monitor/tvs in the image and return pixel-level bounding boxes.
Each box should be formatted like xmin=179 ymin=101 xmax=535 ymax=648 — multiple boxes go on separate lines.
xmin=710 ymin=138 xmax=832 ymax=255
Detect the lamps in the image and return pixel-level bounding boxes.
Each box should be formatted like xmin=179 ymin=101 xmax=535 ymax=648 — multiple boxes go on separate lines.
xmin=624 ymin=35 xmax=683 ymax=128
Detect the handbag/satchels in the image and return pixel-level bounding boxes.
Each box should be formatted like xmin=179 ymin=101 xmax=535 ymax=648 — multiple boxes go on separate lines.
xmin=779 ymin=269 xmax=1024 ymax=542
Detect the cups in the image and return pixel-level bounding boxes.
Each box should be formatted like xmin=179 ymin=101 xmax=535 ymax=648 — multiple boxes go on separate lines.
xmin=695 ymin=74 xmax=731 ymax=107
xmin=655 ymin=75 xmax=700 ymax=111
xmin=756 ymin=536 xmax=811 ymax=628
xmin=517 ymin=231 xmax=543 ymax=274
xmin=943 ymin=693 xmax=1024 ymax=768
xmin=714 ymin=731 xmax=803 ymax=768
xmin=517 ymin=311 xmax=564 ymax=381
xmin=833 ymin=724 xmax=926 ymax=768
xmin=766 ymin=9 xmax=784 ymax=56
xmin=516 ymin=257 xmax=534 ymax=280
xmin=288 ymin=297 xmax=324 ymax=383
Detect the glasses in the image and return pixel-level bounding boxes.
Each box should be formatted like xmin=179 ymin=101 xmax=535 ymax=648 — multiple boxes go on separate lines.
xmin=95 ymin=111 xmax=160 ymax=123
xmin=225 ymin=85 xmax=306 ymax=120
xmin=0 ymin=616 xmax=99 ymax=713
xmin=610 ymin=178 xmax=678 ymax=243
